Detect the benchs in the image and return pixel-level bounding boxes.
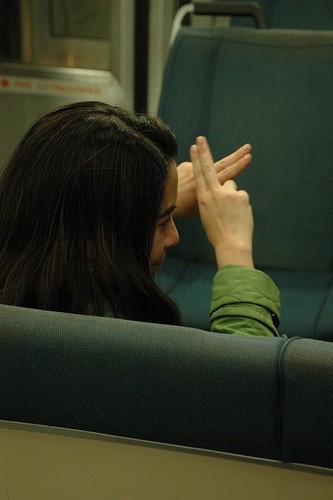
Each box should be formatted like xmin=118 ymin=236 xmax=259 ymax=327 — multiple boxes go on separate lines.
xmin=146 ymin=26 xmax=333 ymax=340
xmin=0 ymin=305 xmax=333 ymax=500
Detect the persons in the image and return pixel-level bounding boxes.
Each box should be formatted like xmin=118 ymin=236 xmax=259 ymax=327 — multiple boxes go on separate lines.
xmin=0 ymin=101 xmax=282 ymax=340
